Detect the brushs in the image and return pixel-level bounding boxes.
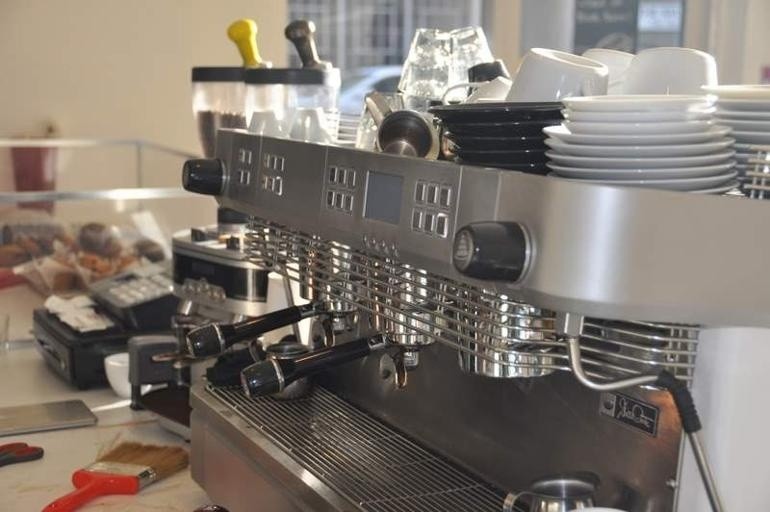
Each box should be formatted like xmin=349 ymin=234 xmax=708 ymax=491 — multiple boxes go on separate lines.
xmin=39 ymin=441 xmax=188 ymax=512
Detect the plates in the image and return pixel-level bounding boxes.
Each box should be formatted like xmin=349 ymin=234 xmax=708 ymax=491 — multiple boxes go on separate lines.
xmin=542 ymin=94 xmax=742 ymax=195
xmin=427 ymin=101 xmax=565 ymax=177
xmin=700 ymin=84 xmax=769 ymax=192
xmin=337 ymin=112 xmax=361 ymax=147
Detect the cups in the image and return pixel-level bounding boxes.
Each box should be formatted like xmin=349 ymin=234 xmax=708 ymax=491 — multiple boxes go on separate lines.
xmin=397 ymin=27 xmax=450 ymax=101
xmin=619 ymin=46 xmax=719 ymax=94
xmin=504 ymin=47 xmax=609 ymax=102
xmin=247 ymin=110 xmax=287 ymax=138
xmin=104 ymin=352 xmax=154 ymax=399
xmin=450 ymin=24 xmax=496 ymax=101
xmin=580 ymin=47 xmax=634 ymax=85
xmin=354 ymin=91 xmax=403 ymax=150
xmin=287 ymin=106 xmax=333 ymax=144
xmin=440 ymin=76 xmax=513 ymax=106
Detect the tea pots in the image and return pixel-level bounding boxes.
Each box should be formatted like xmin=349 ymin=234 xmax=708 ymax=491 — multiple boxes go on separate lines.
xmin=502 ymin=470 xmax=603 ymax=512
xmin=264 ymin=332 xmax=310 ymax=401
xmin=364 ymin=89 xmax=443 ymax=161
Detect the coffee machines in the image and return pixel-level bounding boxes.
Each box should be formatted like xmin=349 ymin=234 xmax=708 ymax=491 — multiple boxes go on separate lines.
xmin=126 ymin=18 xmax=343 ymax=443
xmin=181 ymin=128 xmax=770 ymax=512
